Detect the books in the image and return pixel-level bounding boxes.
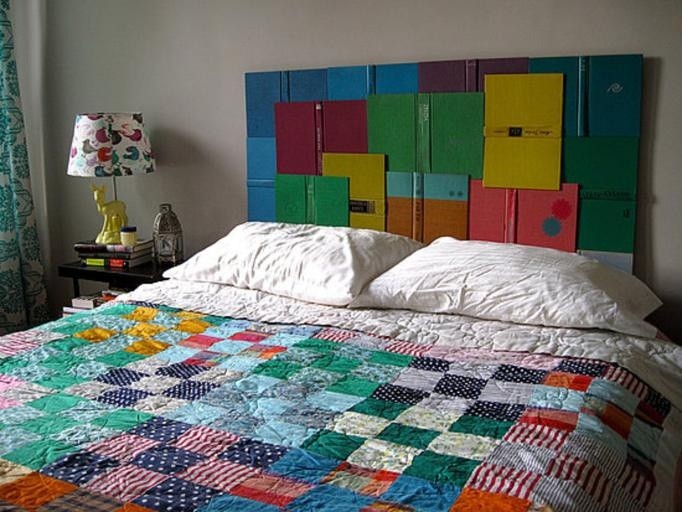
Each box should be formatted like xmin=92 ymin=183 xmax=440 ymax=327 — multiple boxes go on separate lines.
xmin=62 ymin=306 xmax=93 ymax=314
xmin=77 ymin=250 xmax=152 ymax=259
xmin=71 ymin=287 xmax=131 ymax=309
xmin=73 ymin=239 xmax=153 ymax=253
xmin=62 ymin=312 xmax=75 ymax=318
xmin=80 ymin=257 xmax=152 ymax=268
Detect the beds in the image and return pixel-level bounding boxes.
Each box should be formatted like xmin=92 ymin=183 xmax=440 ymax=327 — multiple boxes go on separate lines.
xmin=0 ymin=53 xmax=681 ymax=512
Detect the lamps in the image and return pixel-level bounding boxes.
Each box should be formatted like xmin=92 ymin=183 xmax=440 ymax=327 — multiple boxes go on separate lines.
xmin=66 ymin=110 xmax=156 ymax=244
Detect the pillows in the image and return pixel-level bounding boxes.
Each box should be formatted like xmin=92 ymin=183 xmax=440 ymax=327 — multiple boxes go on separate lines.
xmin=356 ymin=234 xmax=665 ymax=340
xmin=163 ymin=221 xmax=428 ymax=309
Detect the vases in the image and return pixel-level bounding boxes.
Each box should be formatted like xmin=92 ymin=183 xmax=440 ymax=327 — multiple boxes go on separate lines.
xmin=152 ymin=202 xmax=183 ymax=270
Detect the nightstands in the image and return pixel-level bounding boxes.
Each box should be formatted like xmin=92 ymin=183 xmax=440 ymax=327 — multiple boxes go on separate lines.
xmin=57 ymin=256 xmax=181 ymax=320
